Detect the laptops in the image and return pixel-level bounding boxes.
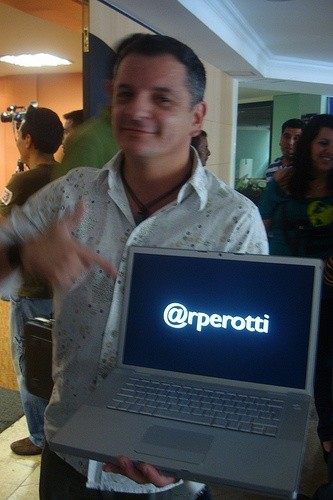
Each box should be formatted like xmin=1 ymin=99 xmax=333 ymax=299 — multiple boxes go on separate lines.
xmin=50 ymin=245 xmax=324 ymax=498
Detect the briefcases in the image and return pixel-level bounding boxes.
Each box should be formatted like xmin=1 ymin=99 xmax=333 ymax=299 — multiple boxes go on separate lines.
xmin=20 ymin=313 xmax=56 ymax=399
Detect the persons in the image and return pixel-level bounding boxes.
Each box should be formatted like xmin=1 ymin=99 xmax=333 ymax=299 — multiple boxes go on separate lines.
xmin=51 ymin=33 xmax=122 ymax=182
xmin=61 ymin=109 xmax=83 ymax=153
xmin=258 ymin=114 xmax=333 ymax=486
xmin=0 ymin=30 xmax=273 ymax=500
xmin=191 ymin=130 xmax=211 ymax=167
xmin=0 ymin=106 xmax=68 ymax=456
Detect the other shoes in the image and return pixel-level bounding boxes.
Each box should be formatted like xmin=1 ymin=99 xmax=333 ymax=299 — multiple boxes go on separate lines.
xmin=320 ymin=440 xmax=333 ymax=464
xmin=10 ymin=437 xmax=44 ymax=455
